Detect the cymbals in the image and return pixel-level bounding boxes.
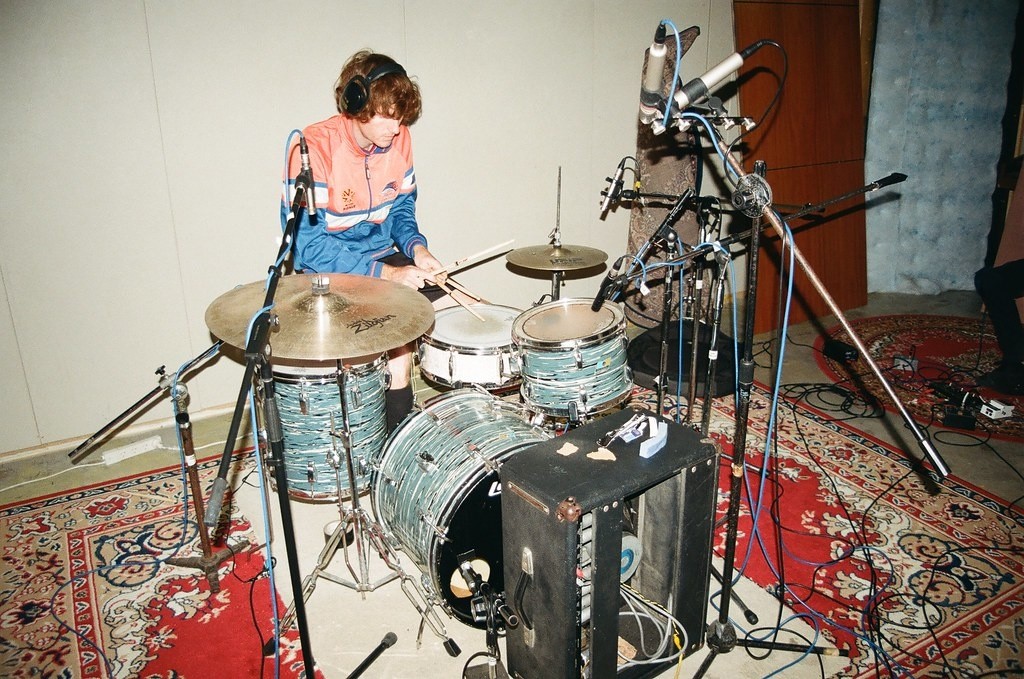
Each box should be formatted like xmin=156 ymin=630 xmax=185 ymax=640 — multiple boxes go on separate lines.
xmin=507 ymin=244 xmax=609 ymax=270
xmin=204 ymin=272 xmax=436 ymax=360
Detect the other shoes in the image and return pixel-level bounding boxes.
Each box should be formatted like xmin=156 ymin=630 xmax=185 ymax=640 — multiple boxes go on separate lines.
xmin=385 ymin=383 xmax=414 ymax=436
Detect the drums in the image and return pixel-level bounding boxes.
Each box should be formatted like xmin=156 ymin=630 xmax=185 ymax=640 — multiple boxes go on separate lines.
xmin=512 ymin=297 xmax=634 ymax=418
xmin=253 ymin=351 xmax=393 ymax=505
xmin=417 ymin=304 xmax=526 ymax=398
xmin=369 ymin=387 xmax=554 ymax=634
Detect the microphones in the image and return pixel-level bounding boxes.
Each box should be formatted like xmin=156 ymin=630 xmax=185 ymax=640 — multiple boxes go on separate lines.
xmin=601 ymin=158 xmax=626 ymax=210
xmin=300 ymin=137 xmax=319 ymax=226
xmin=467 ymin=583 xmax=491 ymax=622
xmin=657 ymin=40 xmax=766 ymax=119
xmin=640 ymin=22 xmax=667 ymax=125
xmin=591 ymin=257 xmax=623 ymax=312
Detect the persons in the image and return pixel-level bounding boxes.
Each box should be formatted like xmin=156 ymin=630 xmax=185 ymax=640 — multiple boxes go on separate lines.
xmin=973 ymin=256 xmax=1024 ymax=394
xmin=280 ymin=50 xmax=479 ymax=442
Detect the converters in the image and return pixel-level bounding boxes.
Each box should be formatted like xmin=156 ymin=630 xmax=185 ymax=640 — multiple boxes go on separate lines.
xmin=822 ymin=340 xmax=858 ymax=364
xmin=942 ymin=405 xmax=976 ymax=431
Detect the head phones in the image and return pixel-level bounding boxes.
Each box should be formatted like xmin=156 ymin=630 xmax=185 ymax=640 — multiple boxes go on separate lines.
xmin=338 ymin=63 xmax=407 ymax=117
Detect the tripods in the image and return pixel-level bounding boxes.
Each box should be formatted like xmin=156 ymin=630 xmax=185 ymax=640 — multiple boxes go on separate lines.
xmin=68 ymin=339 xmax=462 ymax=658
xmin=690 ymin=95 xmax=954 ymax=679
